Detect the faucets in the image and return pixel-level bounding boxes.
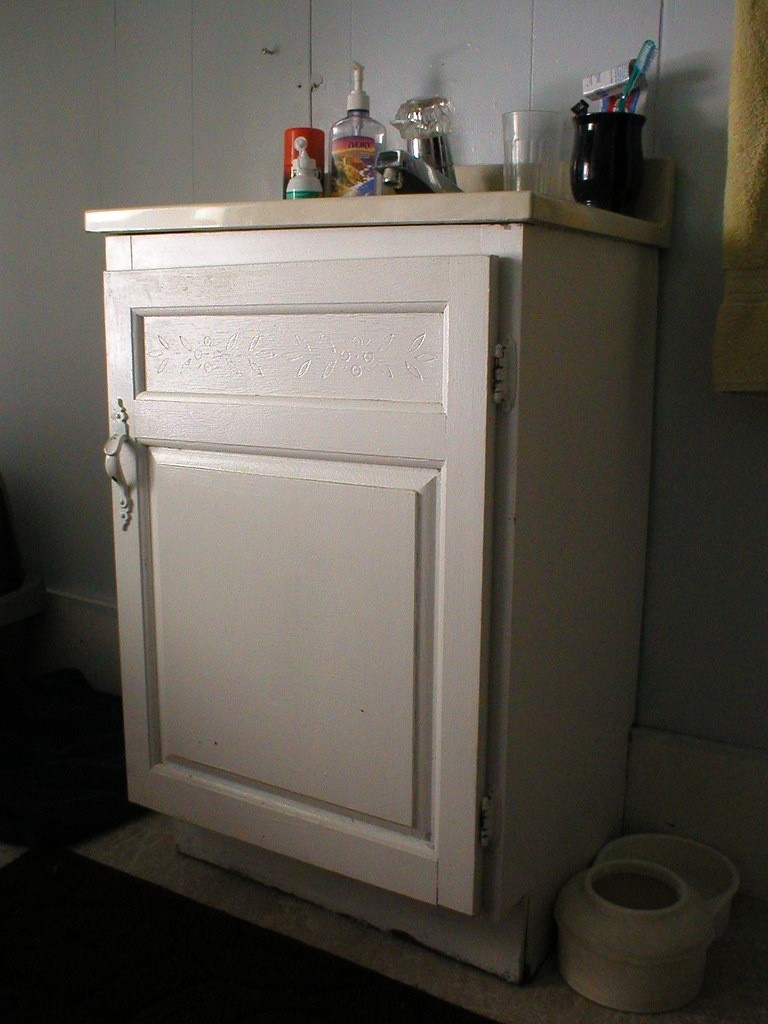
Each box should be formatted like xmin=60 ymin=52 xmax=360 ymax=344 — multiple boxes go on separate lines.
xmin=376 ymin=96 xmax=465 ymax=195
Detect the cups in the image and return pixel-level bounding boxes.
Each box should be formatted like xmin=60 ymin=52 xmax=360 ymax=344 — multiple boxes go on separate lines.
xmin=282 ymin=125 xmax=325 ymax=200
xmin=501 ymin=110 xmax=560 ymax=197
xmin=570 ymin=112 xmax=647 ymax=216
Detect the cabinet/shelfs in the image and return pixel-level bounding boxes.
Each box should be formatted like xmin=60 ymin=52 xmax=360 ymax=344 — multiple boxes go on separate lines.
xmin=86 ymin=157 xmax=674 ymax=982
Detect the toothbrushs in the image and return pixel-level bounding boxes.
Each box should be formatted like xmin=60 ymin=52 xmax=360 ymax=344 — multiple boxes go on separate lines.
xmin=615 ymin=39 xmax=658 ymax=114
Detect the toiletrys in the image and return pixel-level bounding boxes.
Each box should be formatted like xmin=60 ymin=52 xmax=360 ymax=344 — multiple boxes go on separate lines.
xmin=599 ymin=58 xmax=649 ymax=115
xmin=282 ymin=60 xmax=388 ymax=199
